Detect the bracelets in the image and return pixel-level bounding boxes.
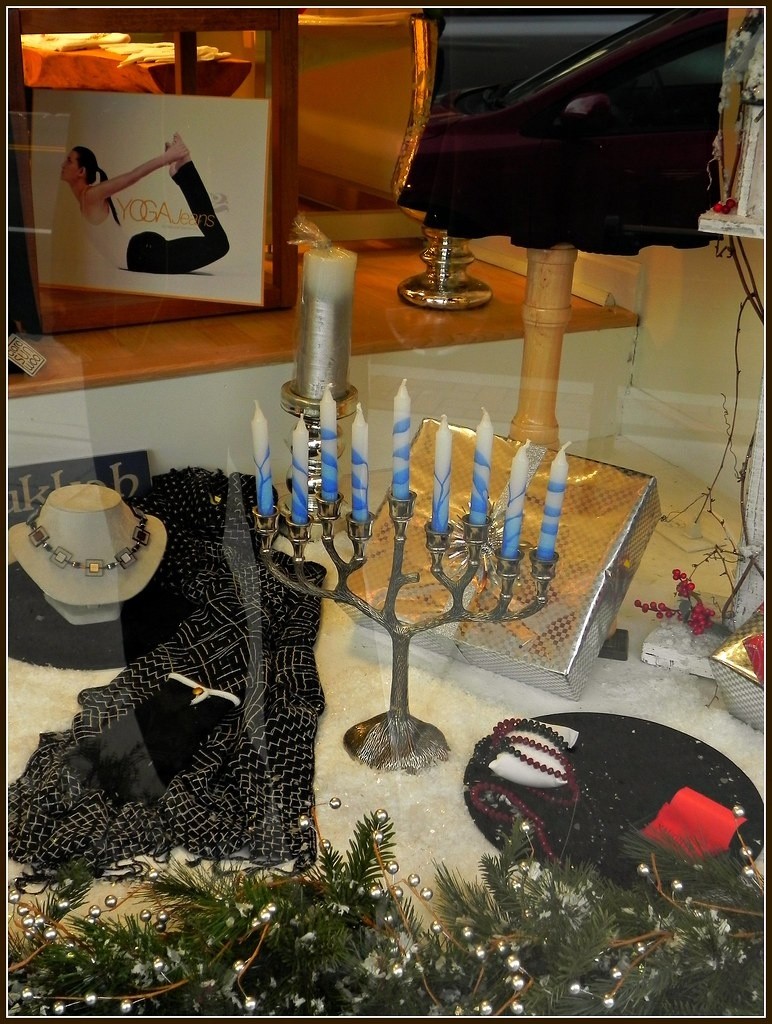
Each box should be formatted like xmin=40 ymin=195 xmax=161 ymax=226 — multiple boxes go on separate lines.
xmin=471 ymin=719 xmax=580 ymax=856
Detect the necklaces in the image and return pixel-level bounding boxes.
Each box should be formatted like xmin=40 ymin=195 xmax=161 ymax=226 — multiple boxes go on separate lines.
xmin=26 ymin=494 xmax=150 ymax=577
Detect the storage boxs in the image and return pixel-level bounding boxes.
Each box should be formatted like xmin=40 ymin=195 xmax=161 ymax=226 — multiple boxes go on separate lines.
xmin=335 ymin=418 xmax=661 ymax=703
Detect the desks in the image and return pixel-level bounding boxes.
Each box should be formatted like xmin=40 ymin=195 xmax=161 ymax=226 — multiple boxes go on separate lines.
xmin=21 ymin=44 xmax=252 ymax=98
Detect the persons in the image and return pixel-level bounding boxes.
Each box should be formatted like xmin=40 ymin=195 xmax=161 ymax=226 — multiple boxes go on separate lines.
xmin=59 ymin=133 xmax=230 ymax=274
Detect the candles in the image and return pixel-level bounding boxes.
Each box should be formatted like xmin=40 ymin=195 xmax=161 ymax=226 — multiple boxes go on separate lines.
xmin=431 ymin=414 xmax=454 ymax=532
xmin=289 ymin=248 xmax=359 ymax=400
xmin=290 ymin=413 xmax=310 ymax=525
xmin=391 ymin=377 xmax=412 ymax=499
xmin=320 ymin=383 xmax=338 ymax=501
xmin=500 ymin=438 xmax=532 ymax=557
xmin=252 ymin=399 xmax=274 ymax=515
xmin=468 ymin=406 xmax=492 ymax=524
xmin=351 ymin=401 xmax=369 ymax=523
xmin=536 ymin=440 xmax=573 ymax=560
